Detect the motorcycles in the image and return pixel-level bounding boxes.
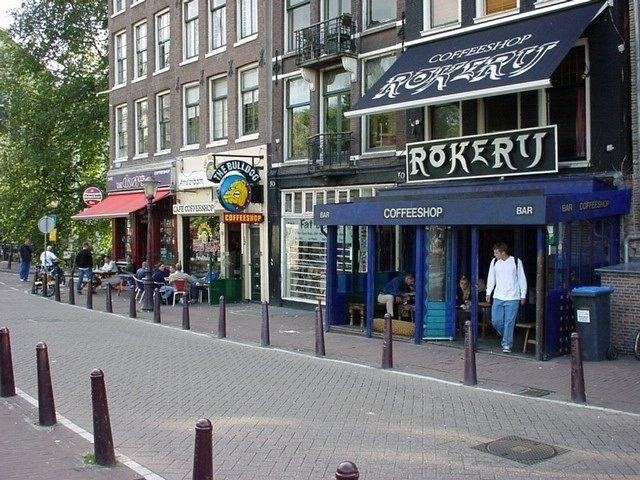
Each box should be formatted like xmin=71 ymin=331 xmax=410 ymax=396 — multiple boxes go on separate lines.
xmin=34 ymin=260 xmax=67 ymax=283
xmin=34 ymin=274 xmax=56 ymax=296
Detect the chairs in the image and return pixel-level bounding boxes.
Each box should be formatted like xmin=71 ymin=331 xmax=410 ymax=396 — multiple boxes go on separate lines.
xmin=41 ymin=262 xmax=200 ymax=307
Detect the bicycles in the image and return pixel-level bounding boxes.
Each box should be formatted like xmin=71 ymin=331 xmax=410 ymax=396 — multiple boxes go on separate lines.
xmin=633 ymin=330 xmax=640 ymax=361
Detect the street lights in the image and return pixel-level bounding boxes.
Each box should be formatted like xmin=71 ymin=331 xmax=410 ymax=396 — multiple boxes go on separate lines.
xmin=142 ymin=175 xmax=161 ymax=313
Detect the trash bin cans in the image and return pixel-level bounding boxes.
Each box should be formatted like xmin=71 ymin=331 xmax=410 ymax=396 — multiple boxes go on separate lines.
xmin=569 ymin=285 xmax=619 ymax=363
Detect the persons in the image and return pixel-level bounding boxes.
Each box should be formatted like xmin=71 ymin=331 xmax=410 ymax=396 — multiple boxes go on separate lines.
xmin=100 ymin=254 xmax=218 ymax=304
xmin=457 ymin=275 xmax=471 ymax=301
xmin=478 ymin=278 xmax=487 ymax=302
xmin=76 ymin=239 xmax=95 ymax=295
xmin=40 ymin=245 xmax=60 ymax=279
xmin=486 ymin=243 xmax=528 ymax=354
xmin=20 ymin=237 xmax=31 ymax=282
xmin=378 ymin=273 xmax=414 ymax=319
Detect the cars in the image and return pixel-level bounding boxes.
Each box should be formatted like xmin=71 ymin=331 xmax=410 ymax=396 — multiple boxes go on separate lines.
xmin=0 ymin=242 xmax=21 ymax=261
